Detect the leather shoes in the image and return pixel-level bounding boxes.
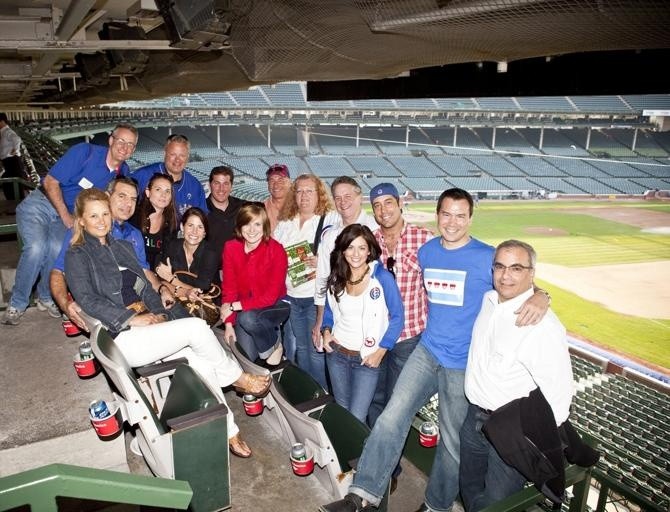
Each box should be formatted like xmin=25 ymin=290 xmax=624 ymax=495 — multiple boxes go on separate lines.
xmin=318 ymin=493 xmax=378 ymax=512
xmin=263 ymin=360 xmax=280 ymax=369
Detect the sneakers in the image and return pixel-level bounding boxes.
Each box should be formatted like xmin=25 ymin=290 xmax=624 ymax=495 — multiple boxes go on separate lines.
xmin=1 ymin=305 xmax=25 ymax=325
xmin=37 ymin=298 xmax=63 ymax=318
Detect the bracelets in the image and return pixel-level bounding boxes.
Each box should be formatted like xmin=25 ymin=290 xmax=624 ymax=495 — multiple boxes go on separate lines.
xmin=158 ymin=284 xmax=167 ymax=295
xmin=322 ymin=328 xmax=330 ymax=335
xmin=170 ymin=276 xmax=175 ymax=283
xmin=535 ymin=289 xmax=552 ymax=306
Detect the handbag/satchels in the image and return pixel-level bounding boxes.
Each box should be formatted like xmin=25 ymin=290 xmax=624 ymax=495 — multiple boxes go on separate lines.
xmin=172 ymin=270 xmax=221 ymax=328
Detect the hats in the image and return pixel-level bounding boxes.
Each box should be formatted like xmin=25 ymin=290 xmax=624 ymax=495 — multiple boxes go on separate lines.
xmin=370 ymin=183 xmax=399 ymax=202
xmin=266 ymin=164 xmax=289 ymax=177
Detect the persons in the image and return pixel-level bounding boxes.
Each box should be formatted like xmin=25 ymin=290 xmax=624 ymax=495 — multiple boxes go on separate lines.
xmin=200 ymin=166 xmax=250 ymax=298
xmin=49 ymin=176 xmax=174 ymax=334
xmin=459 ymin=239 xmax=570 ymax=512
xmin=367 ymin=183 xmax=435 ymax=478
xmin=64 ymin=188 xmax=272 ymax=458
xmin=261 ymin=164 xmax=291 ymax=239
xmin=130 ymin=134 xmax=209 ymax=231
xmin=317 ymin=186 xmax=551 ymax=512
xmin=271 ymin=174 xmax=333 ymax=394
xmin=0 ymin=112 xmax=25 ymax=216
xmin=219 ymin=203 xmax=291 ymax=367
xmin=319 ymin=223 xmax=405 ymax=431
xmin=154 ymin=207 xmax=220 ymax=304
xmin=312 ymin=176 xmax=381 ymax=352
xmin=130 ymin=172 xmax=180 ymax=274
xmin=1 ymin=122 xmax=139 ymax=326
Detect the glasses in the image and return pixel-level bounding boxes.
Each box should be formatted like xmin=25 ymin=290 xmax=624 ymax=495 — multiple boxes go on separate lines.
xmin=387 ymin=257 xmax=395 ymax=279
xmin=112 ymin=135 xmax=135 ymax=151
xmin=491 ymin=263 xmax=532 ymax=273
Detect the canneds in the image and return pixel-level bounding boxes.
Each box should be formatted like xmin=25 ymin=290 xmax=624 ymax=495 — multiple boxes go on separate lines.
xmin=79 ymin=341 xmax=93 ymax=361
xmin=291 ymin=443 xmax=307 ymax=461
xmin=62 ymin=313 xmax=69 ymax=321
xmin=422 ymin=423 xmax=436 ymax=435
xmin=88 ymin=400 xmax=110 ymax=419
xmin=244 ymin=394 xmax=257 ymax=402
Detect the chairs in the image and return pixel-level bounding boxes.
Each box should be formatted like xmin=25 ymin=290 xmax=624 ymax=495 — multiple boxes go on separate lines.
xmin=50 ymin=268 xmax=189 ymax=422
xmin=270 ymin=380 xmax=397 ymax=501
xmin=416 ymin=391 xmax=439 ymax=426
xmin=229 ymin=332 xmax=332 ymax=416
xmin=90 ymin=324 xmax=232 ymax=512
xmin=569 ymin=351 xmax=669 ymax=511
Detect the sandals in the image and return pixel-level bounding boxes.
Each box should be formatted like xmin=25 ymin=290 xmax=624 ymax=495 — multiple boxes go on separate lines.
xmin=229 ymin=431 xmax=252 ymax=457
xmin=234 ymin=373 xmax=272 ymax=398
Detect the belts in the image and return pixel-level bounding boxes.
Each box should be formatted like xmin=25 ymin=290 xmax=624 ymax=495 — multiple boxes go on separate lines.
xmin=330 ymin=342 xmax=360 ymax=356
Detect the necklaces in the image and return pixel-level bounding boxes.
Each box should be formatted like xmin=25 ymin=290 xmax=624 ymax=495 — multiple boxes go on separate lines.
xmin=441 ymin=239 xmax=444 ymax=247
xmin=346 ymin=266 xmax=369 ymax=286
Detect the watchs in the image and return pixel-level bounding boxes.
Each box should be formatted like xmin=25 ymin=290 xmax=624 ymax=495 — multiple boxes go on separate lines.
xmin=230 ymin=302 xmax=234 ymax=312
